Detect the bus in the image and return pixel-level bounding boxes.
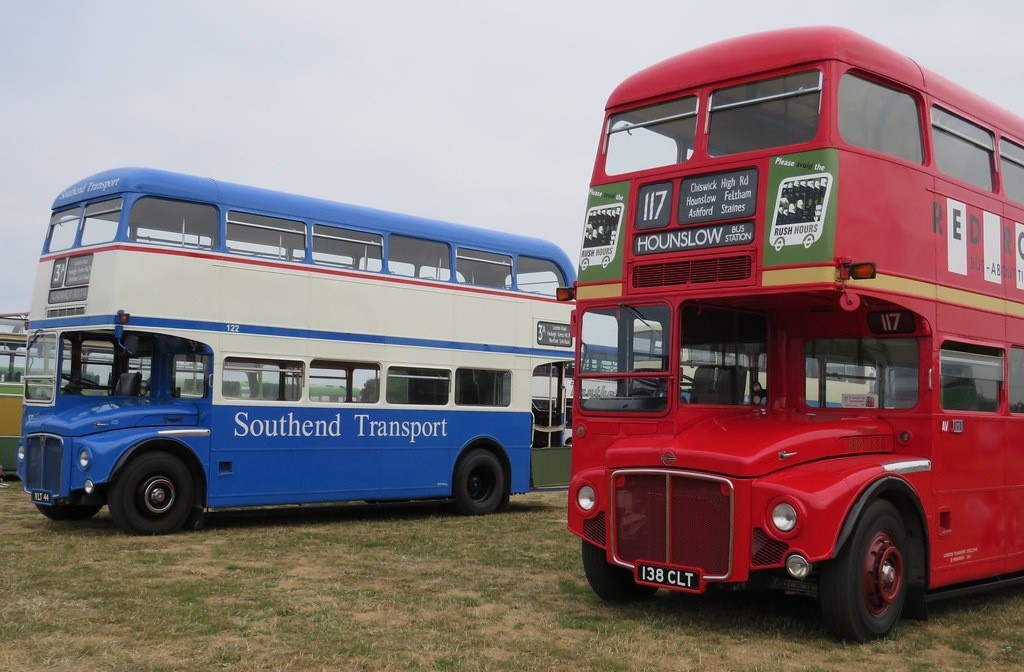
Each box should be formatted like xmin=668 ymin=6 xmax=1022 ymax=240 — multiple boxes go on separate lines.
xmin=12 ymin=166 xmax=579 ymax=538
xmin=1 ymin=310 xmax=31 ymax=483
xmin=555 ymin=24 xmax=1020 ymax=645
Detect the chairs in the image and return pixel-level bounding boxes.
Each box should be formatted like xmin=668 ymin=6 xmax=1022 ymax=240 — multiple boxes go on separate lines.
xmin=411 ymin=380 xmax=438 ymax=404
xmin=183 ymin=379 xmax=298 ymax=401
xmin=84 ymin=375 xmax=99 ymax=384
xmin=689 ymin=365 xmax=747 ymax=405
xmin=10 ymin=371 xmax=20 ymax=383
xmin=112 ymin=373 xmax=142 ymax=395
xmin=3 ymin=371 xmax=12 ymax=383
xmin=941 ymin=365 xmax=977 ymax=410
